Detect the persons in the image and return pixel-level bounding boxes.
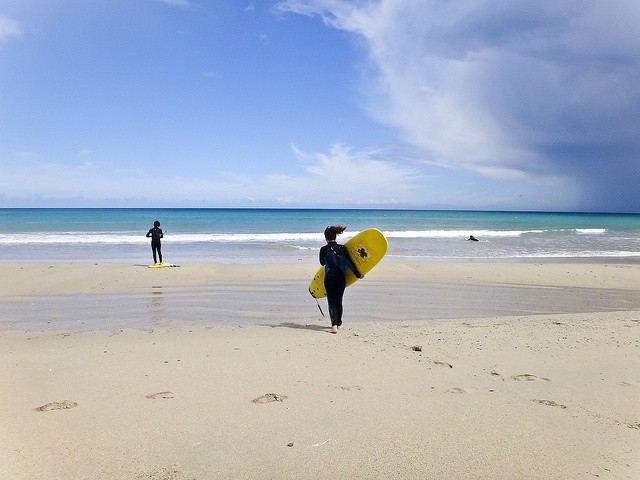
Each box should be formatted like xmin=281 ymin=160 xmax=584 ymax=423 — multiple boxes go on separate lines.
xmin=318 ymin=225 xmax=365 ymax=333
xmin=467 ymin=235 xmax=480 ymax=241
xmin=145 ymin=220 xmax=164 ymax=264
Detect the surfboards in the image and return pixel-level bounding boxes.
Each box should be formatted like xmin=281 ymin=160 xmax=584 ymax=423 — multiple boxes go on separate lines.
xmin=309 ymin=227 xmax=389 ymax=299
xmin=147 ymin=263 xmax=179 ymax=269
